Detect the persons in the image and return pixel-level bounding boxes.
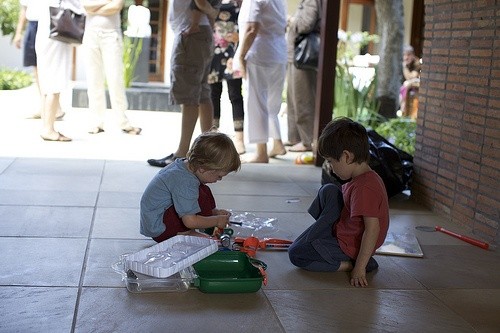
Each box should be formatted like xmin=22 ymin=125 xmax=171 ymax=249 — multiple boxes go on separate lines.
xmin=147 ymin=0 xmax=222 ymax=168
xmin=282 ymin=0 xmax=323 ymax=151
xmin=82 ymin=0 xmax=142 ymax=136
xmin=400 ymin=44 xmax=421 ymax=86
xmin=287 ymin=116 xmax=390 ymax=288
xmin=179 ymin=0 xmax=222 ymax=38
xmin=13 ymin=0 xmax=73 ymax=141
xmin=209 ymin=0 xmax=287 ymax=165
xmin=139 ymin=132 xmax=242 ymax=243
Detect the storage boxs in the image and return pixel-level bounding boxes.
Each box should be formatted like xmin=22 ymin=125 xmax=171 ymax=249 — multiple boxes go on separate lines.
xmin=109 ymin=251 xmax=268 ymax=294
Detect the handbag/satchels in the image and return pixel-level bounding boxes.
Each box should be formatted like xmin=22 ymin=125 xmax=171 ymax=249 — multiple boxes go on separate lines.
xmin=49 ymin=0 xmax=86 ymax=45
xmin=293 ymin=31 xmax=320 ymax=70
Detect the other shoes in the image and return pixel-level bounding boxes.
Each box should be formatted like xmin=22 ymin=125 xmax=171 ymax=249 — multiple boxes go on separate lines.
xmin=88 ymin=127 xmax=104 ymax=134
xmin=147 ymin=153 xmax=186 ymax=167
xmin=367 ymin=257 xmax=379 ymax=271
xmin=122 ymin=127 xmax=143 ymax=135
xmin=43 ymin=131 xmax=73 ymax=141
